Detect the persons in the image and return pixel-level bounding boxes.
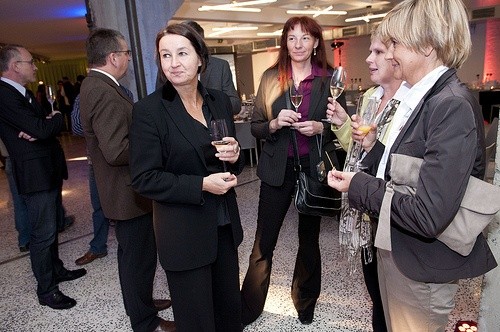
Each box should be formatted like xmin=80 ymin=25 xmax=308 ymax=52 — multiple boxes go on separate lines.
xmin=239 ymin=16 xmax=347 ymax=326
xmin=154 ymin=20 xmax=241 ymax=115
xmin=71 ymin=83 xmax=134 ymax=264
xmin=0 ymin=138 xmax=75 ymax=252
xmin=73 ymin=75 xmax=85 ymax=97
xmin=33 ymin=75 xmax=72 ymax=133
xmin=0 ymin=45 xmax=86 ymax=309
xmin=80 ymin=24 xmax=243 ymax=332
xmin=325 ymin=0 xmax=498 ymax=332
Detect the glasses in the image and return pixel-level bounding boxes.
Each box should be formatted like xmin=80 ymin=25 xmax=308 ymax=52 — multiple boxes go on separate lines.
xmin=107 ymin=50 xmax=132 ymax=56
xmin=15 ymin=59 xmax=36 ymax=65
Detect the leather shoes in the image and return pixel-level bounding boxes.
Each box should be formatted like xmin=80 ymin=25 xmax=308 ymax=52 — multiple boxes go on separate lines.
xmin=152 ymin=299 xmax=171 ymax=310
xmin=75 ymin=251 xmax=107 ymax=266
xmin=152 ymin=318 xmax=177 ymax=332
xmin=38 ymin=291 xmax=76 ymax=309
xmin=58 ymin=269 xmax=86 ymax=283
xmin=298 ymin=307 xmax=313 ymax=324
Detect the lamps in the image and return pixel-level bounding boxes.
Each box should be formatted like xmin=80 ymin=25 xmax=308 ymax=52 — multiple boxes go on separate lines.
xmin=285 ymin=10 xmax=348 ymax=16
xmin=344 ymin=12 xmax=387 ymax=25
xmin=198 ymin=0 xmax=278 ymax=12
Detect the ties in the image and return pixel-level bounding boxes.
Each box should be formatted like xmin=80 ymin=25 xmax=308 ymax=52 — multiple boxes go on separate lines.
xmin=25 ymin=92 xmax=30 ymax=101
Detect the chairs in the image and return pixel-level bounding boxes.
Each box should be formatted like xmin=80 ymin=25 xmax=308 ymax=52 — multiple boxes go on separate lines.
xmin=483 ymin=118 xmax=498 ymax=162
xmin=233 ymin=121 xmax=259 ymax=170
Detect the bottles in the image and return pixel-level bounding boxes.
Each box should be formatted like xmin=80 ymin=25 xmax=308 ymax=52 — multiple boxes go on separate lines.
xmin=476 ymin=73 xmax=494 ymax=90
xmin=350 ymin=77 xmax=363 ymax=91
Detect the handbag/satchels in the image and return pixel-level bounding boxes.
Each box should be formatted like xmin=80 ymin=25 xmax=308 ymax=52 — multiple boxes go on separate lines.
xmin=294 ymin=172 xmax=341 ymax=217
xmin=374 ymin=153 xmax=500 ymax=256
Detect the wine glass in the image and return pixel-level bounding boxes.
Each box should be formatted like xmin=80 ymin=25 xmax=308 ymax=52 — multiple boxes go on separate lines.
xmin=346 ymin=93 xmax=377 ymax=170
xmin=210 ymin=118 xmax=229 ymax=173
xmin=321 ymin=66 xmax=347 ymax=125
xmin=289 ymin=79 xmax=303 ymax=113
xmin=45 ymin=84 xmax=57 ymax=120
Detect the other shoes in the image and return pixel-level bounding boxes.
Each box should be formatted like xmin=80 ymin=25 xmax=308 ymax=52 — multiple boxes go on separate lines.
xmin=20 ymin=242 xmax=30 ymax=252
xmin=58 ymin=215 xmax=75 ymax=233
